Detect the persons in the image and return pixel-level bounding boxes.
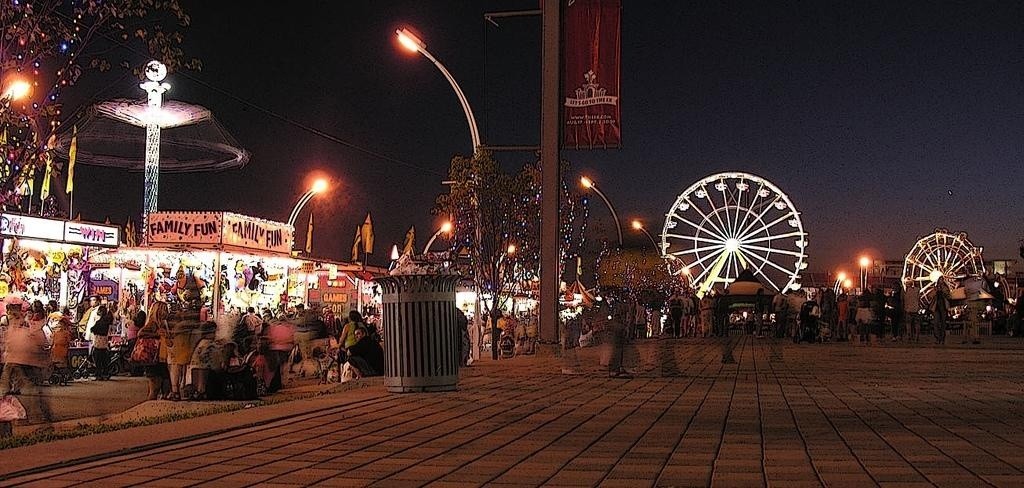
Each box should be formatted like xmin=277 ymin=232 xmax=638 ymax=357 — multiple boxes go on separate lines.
xmin=1 ymin=292 xmax=383 ymax=439
xmin=457 ymin=275 xmax=1016 ymax=380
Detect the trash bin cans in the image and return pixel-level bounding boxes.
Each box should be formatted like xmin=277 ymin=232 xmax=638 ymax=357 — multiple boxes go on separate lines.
xmin=372 ymin=273 xmax=465 ymax=394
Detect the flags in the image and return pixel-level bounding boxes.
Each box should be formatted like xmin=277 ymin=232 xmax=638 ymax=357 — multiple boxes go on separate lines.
xmin=40 ymin=124 xmax=55 ymax=201
xmin=351 ymin=225 xmax=361 ymax=262
xmin=65 ymin=126 xmax=78 ymax=194
xmin=360 ymin=212 xmax=375 ymax=254
xmin=0 ymin=128 xmax=9 ymax=184
xmin=403 ymin=227 xmax=417 ymax=255
xmin=18 ymin=132 xmax=38 ymax=197
xmin=305 ymin=212 xmax=314 ymax=254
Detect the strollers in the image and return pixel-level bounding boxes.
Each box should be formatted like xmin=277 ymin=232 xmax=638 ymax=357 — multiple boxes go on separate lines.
xmin=34 ymin=348 xmax=125 ymax=387
xmin=499 ymin=334 xmax=516 ymax=360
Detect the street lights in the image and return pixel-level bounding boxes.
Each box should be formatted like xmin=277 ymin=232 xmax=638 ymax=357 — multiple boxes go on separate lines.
xmin=578 ymin=173 xmax=625 ymax=246
xmin=833 ymin=271 xmax=849 ymax=294
xmin=496 ymin=244 xmax=517 ymax=267
xmin=424 ymin=218 xmax=454 ymax=254
xmin=629 ymin=218 xmax=663 ymax=255
xmin=855 ymin=254 xmax=870 ymax=290
xmin=391 ymin=24 xmax=485 ymax=362
xmin=288 ymin=172 xmax=332 ymax=227
